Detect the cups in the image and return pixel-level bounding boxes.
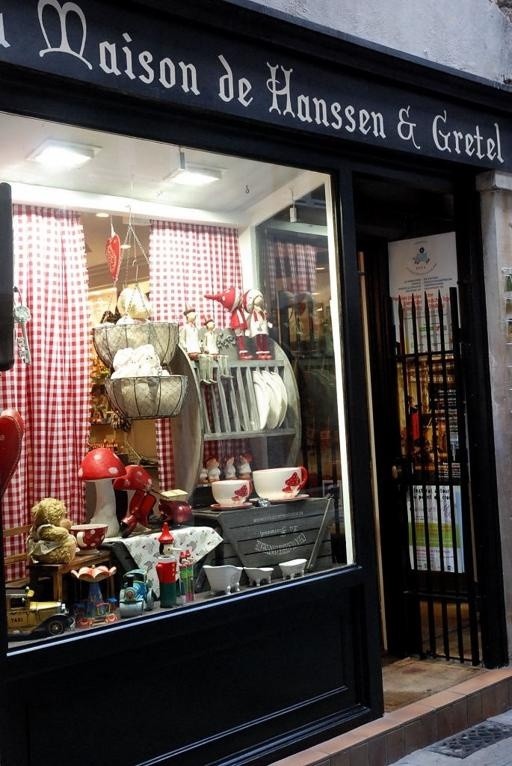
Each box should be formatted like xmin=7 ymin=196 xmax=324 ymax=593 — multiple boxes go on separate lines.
xmin=210 ymin=466 xmax=308 ymax=506
xmin=68 ymin=524 xmax=108 ymax=556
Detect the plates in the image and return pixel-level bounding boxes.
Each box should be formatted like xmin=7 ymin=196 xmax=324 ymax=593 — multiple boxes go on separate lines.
xmin=262 ymin=495 xmax=314 ymax=505
xmin=207 ymin=502 xmax=254 ymax=510
xmin=240 ymin=370 xmax=288 ymax=435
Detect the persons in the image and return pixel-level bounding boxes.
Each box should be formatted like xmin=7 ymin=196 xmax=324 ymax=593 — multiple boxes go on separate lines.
xmin=202 ymin=286 xmax=255 ymax=361
xmin=244 ymin=287 xmax=279 ymax=361
xmin=198 ymin=451 xmax=256 ymax=485
xmin=203 ymin=319 xmax=237 ymax=382
xmin=179 ymin=308 xmax=216 ymax=385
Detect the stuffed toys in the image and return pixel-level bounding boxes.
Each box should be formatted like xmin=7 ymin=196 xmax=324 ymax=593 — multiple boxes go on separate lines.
xmin=112 ymin=344 xmax=169 ymax=378
xmin=114 ymin=289 xmax=152 ymax=325
xmin=25 ymin=498 xmax=77 ymax=564
